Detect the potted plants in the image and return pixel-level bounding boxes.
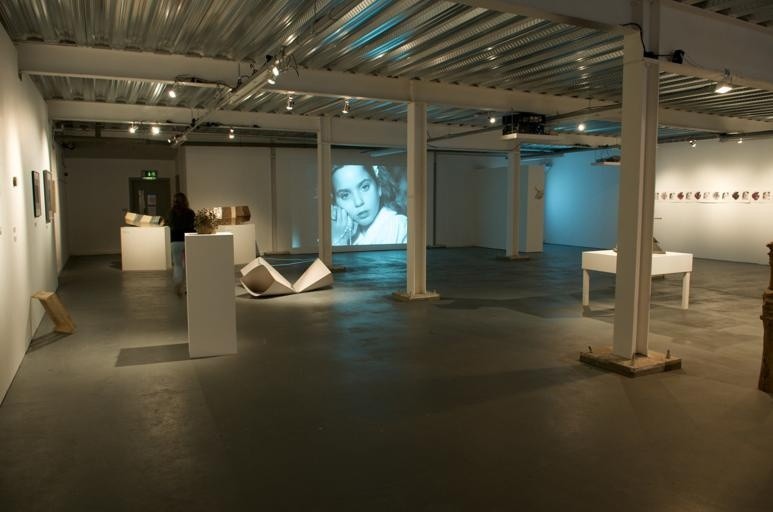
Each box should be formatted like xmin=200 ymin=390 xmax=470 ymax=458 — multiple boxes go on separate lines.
xmin=194 ymin=208 xmax=219 ymax=234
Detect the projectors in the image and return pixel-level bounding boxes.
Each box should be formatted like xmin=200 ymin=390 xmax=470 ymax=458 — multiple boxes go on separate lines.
xmin=501 ymin=112 xmax=547 ymax=134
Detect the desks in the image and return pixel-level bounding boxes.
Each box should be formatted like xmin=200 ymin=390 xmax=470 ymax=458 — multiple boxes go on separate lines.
xmin=582 ymin=250 xmax=694 ymax=309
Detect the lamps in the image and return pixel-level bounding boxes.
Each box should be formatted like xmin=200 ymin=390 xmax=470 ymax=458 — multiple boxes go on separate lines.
xmin=338 ymin=97 xmax=353 ymax=115
xmin=168 ymin=78 xmax=180 ymax=97
xmin=267 ymin=47 xmax=290 ymax=86
xmin=714 ymin=68 xmax=734 ymax=94
xmin=282 ymin=92 xmax=296 ymax=111
xmin=128 ymin=120 xmax=140 ymax=135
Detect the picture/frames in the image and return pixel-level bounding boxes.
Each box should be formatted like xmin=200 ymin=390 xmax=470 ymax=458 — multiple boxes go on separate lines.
xmin=44 ymin=171 xmax=54 ymax=222
xmin=31 ymin=170 xmax=43 ymax=218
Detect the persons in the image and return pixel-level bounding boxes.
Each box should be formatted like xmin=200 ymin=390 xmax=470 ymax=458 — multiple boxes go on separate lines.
xmin=162 ymin=192 xmax=197 ymax=298
xmin=331 ymin=164 xmax=406 ymax=246
